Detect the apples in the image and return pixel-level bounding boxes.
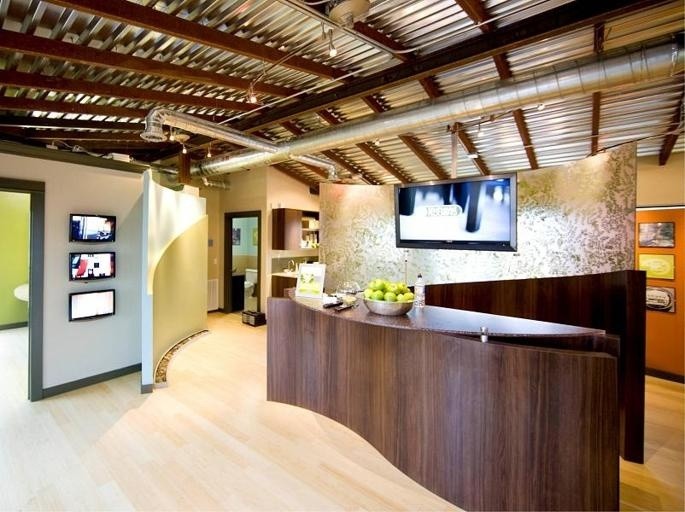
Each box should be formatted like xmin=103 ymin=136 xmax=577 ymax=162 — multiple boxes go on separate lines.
xmin=384 ymin=280 xmax=390 ymax=288
xmin=404 ymin=292 xmax=414 ymax=300
xmin=388 ymin=283 xmax=398 ymax=293
xmin=399 ymin=283 xmax=407 ymax=293
xmin=384 ymin=292 xmax=397 ymax=301
xmin=397 ymin=294 xmax=404 ymax=301
xmin=406 ymin=288 xmax=410 ymax=293
xmin=369 ymin=279 xmax=384 ymax=291
xmin=364 ymin=288 xmax=372 ymax=297
xmin=372 ymin=291 xmax=383 ymax=300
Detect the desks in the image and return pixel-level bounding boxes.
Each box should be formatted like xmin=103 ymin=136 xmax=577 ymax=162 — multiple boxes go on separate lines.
xmin=232 ymin=274 xmax=245 ymax=311
xmin=272 ymin=273 xmax=297 ymax=297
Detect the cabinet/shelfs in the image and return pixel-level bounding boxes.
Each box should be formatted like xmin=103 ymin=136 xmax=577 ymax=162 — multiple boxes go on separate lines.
xmin=272 ymin=208 xmax=320 ymax=250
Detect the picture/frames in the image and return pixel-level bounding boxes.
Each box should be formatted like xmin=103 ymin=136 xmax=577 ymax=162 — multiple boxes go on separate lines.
xmin=646 ymin=286 xmax=676 ymax=313
xmin=295 ymin=263 xmax=326 ymax=299
xmin=638 ymin=222 xmax=675 ymax=248
xmin=638 ymin=254 xmax=674 ymax=280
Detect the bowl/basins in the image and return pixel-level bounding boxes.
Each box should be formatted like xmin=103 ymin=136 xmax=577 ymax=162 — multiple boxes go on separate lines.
xmin=356 ymin=291 xmax=418 ymax=315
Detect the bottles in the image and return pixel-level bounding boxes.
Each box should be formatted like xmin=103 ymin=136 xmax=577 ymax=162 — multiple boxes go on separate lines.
xmin=414 ymin=273 xmax=425 ymax=306
xmin=480 ymin=327 xmax=489 ymax=343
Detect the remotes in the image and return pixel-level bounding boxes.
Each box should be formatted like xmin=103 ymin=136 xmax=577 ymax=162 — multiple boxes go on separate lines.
xmin=335 ymin=304 xmax=353 ymax=312
xmin=322 ymin=301 xmax=341 ymax=309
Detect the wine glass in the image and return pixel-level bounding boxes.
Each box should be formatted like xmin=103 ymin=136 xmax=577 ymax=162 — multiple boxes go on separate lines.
xmin=337 ymin=280 xmax=360 ymax=306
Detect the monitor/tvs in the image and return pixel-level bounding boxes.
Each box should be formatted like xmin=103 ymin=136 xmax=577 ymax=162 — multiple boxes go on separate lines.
xmin=394 ymin=170 xmax=518 ymax=252
